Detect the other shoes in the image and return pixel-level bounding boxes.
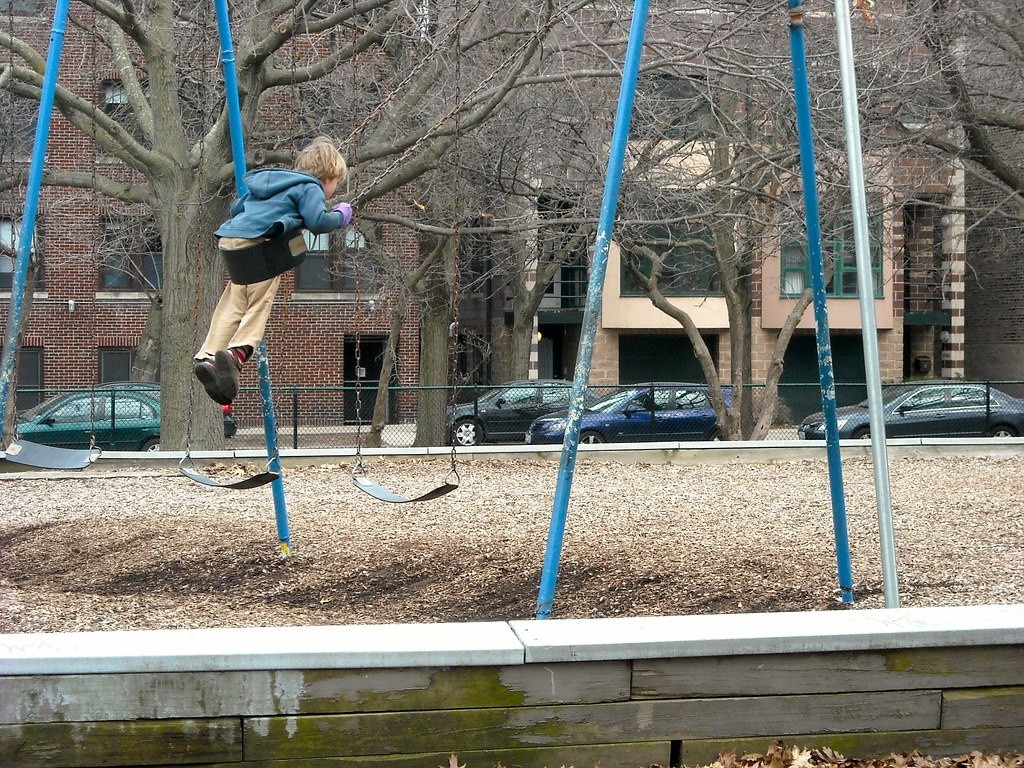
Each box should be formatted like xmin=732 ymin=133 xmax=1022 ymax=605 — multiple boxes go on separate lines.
xmin=215 ymin=349 xmax=242 ymax=400
xmin=195 ymin=359 xmax=232 ymax=406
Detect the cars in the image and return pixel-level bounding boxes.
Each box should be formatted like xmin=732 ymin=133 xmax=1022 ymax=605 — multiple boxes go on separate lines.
xmin=797 ymin=380 xmax=1024 ymax=439
xmin=106 ymin=382 xmax=238 ymax=439
xmin=524 ymin=381 xmax=730 ymax=445
xmin=15 ymin=385 xmax=160 ymax=452
xmin=445 ymin=379 xmax=602 ymax=446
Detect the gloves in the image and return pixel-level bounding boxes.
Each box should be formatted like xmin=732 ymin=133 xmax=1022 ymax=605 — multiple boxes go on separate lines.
xmin=332 ymin=202 xmax=352 ymax=229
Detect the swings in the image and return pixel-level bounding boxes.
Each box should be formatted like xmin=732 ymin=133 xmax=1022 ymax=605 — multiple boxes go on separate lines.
xmin=177 ymin=1 xmax=301 ymax=491
xmin=221 ymin=0 xmax=578 ymax=286
xmin=2 ymin=6 xmax=102 ymax=469
xmin=350 ymin=0 xmax=462 ymax=505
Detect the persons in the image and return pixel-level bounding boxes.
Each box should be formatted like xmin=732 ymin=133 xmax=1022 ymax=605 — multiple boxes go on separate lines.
xmin=193 ymin=136 xmax=352 ymax=405
